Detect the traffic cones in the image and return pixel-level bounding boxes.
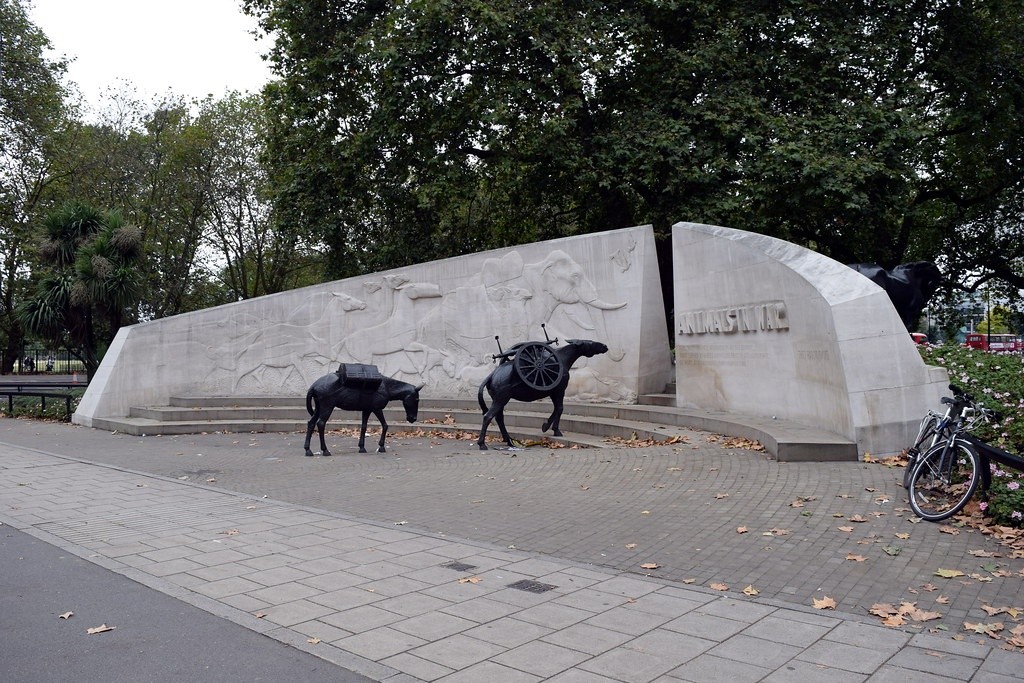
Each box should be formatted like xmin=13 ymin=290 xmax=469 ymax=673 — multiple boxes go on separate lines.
xmin=71 ymin=371 xmax=78 ymax=384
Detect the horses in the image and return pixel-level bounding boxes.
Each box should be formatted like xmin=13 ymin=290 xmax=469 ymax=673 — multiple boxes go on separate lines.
xmin=477 ymin=338 xmax=608 ymax=450
xmin=303 ymin=371 xmax=425 ymax=456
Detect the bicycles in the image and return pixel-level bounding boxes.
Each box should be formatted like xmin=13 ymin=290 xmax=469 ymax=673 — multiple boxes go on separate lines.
xmin=901 ymin=382 xmax=1007 ymax=522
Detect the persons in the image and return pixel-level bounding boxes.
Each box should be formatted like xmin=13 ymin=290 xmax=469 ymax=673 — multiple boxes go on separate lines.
xmin=46 ymin=356 xmax=56 ymax=372
xmin=24 ymin=357 xmax=30 ymax=372
xmin=29 ymin=359 xmax=35 ymax=372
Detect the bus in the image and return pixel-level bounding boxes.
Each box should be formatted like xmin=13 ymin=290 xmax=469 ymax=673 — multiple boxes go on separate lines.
xmin=964 ymin=333 xmax=1018 ymax=352
